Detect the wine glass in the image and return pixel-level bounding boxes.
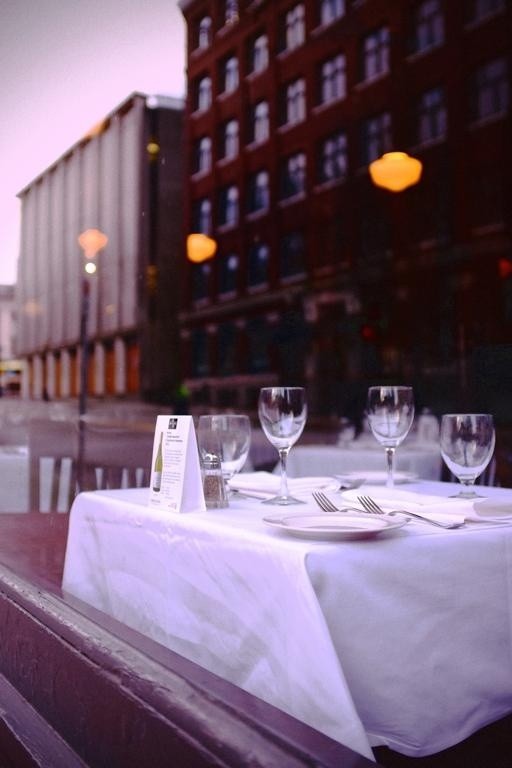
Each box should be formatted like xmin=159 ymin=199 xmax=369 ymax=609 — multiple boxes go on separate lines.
xmin=367 ymin=385 xmax=495 ymax=498
xmin=197 ymin=386 xmax=307 ymax=504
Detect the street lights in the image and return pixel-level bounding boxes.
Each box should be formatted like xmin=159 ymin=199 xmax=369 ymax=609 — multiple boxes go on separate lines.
xmin=76 ymin=231 xmax=109 ymax=467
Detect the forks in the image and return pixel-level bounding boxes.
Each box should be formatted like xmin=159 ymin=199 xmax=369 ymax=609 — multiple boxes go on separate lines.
xmin=311 ymin=490 xmax=466 ymax=533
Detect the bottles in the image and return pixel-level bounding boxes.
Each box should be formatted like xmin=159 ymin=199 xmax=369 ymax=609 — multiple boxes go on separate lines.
xmin=152 ymin=432 xmax=163 ymax=492
xmin=200 ymin=454 xmax=229 ymax=510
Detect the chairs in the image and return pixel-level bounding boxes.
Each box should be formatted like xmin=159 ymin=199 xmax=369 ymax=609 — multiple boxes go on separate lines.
xmin=25 ymin=418 xmax=155 ymax=512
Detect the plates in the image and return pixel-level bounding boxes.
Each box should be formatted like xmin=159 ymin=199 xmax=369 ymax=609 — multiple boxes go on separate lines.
xmin=263 ymin=510 xmax=407 ymax=541
xmin=335 ymin=470 xmax=418 ymax=485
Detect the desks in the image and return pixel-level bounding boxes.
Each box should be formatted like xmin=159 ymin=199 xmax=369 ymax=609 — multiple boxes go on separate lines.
xmin=54 ymin=436 xmax=512 ymax=756
